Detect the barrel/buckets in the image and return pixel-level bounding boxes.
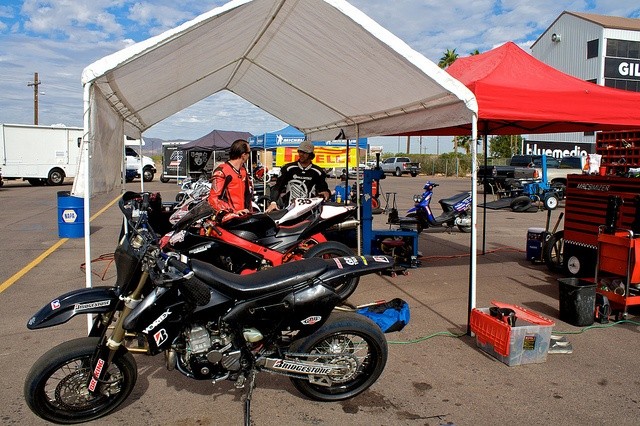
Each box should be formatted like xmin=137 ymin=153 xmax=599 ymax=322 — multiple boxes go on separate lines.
xmin=526 ymin=227 xmax=546 ymax=260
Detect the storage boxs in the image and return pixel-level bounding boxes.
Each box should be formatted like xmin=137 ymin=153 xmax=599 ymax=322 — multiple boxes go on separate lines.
xmin=470 ymin=301 xmax=556 ymax=367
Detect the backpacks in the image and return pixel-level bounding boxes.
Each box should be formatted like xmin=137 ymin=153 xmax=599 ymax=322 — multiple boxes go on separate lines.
xmin=357 ymin=297 xmax=410 ymax=334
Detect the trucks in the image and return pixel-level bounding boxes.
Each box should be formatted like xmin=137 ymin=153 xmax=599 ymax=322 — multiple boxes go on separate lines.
xmin=0 ymin=124 xmax=156 ymax=186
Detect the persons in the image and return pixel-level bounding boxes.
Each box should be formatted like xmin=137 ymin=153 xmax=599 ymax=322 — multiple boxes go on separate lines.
xmin=339 ymin=168 xmax=347 ymax=182
xmin=323 ymin=168 xmax=333 ymax=174
xmin=264 ymin=140 xmax=332 ymax=215
xmin=208 ymin=138 xmax=254 ymax=216
xmin=527 ymin=162 xmax=540 ymax=178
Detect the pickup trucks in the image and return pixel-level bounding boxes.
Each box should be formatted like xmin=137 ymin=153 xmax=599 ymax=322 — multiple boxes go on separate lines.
xmin=477 ymin=155 xmax=582 ymax=196
xmin=514 ymin=156 xmax=600 ymax=199
xmin=381 ymin=157 xmax=421 ymax=177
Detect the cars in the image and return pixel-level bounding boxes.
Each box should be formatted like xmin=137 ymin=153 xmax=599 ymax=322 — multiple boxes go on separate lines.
xmin=349 ymin=165 xmax=369 ymax=179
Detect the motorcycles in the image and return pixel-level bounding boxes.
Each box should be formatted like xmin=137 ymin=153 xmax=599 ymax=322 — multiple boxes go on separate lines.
xmin=169 ymin=177 xmax=358 ymax=228
xmin=118 ymin=190 xmax=359 ymax=300
xmin=403 ymin=181 xmax=472 ymax=234
xmin=24 ymin=192 xmax=395 ymax=426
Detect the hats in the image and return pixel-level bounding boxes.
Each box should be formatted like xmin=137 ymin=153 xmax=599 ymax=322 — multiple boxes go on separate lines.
xmin=297 ymin=140 xmax=314 ymax=154
